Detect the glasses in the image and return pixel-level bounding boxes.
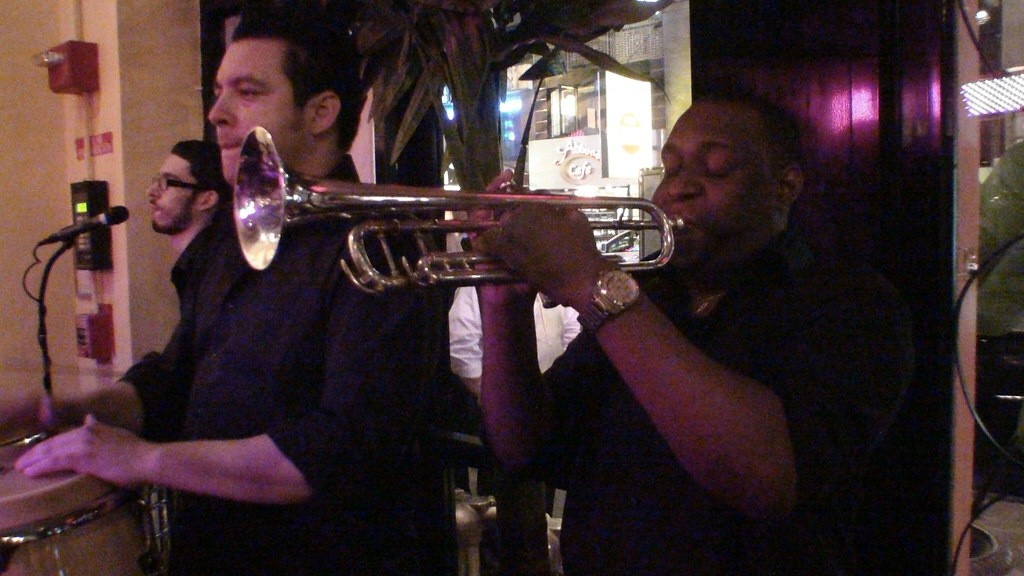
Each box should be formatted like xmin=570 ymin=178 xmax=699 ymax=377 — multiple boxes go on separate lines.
xmin=152 ymin=174 xmax=203 ymax=190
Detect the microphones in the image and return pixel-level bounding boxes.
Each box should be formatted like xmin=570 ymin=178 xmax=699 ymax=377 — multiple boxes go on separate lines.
xmin=38 ymin=206 xmax=129 ymax=246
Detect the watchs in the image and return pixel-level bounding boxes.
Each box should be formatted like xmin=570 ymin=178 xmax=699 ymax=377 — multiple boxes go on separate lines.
xmin=577 ymin=265 xmax=640 ymax=335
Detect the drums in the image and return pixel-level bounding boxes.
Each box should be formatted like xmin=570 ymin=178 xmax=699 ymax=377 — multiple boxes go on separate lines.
xmin=0 ymin=426 xmax=170 ymax=576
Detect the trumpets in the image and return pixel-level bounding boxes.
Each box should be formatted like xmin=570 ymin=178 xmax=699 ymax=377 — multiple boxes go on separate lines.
xmin=232 ymin=125 xmax=687 ymax=300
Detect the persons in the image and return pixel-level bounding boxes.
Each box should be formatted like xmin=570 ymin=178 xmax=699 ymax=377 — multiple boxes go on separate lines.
xmin=0 ymin=4 xmax=581 ymax=576
xmin=469 ymin=92 xmax=916 ymax=576
